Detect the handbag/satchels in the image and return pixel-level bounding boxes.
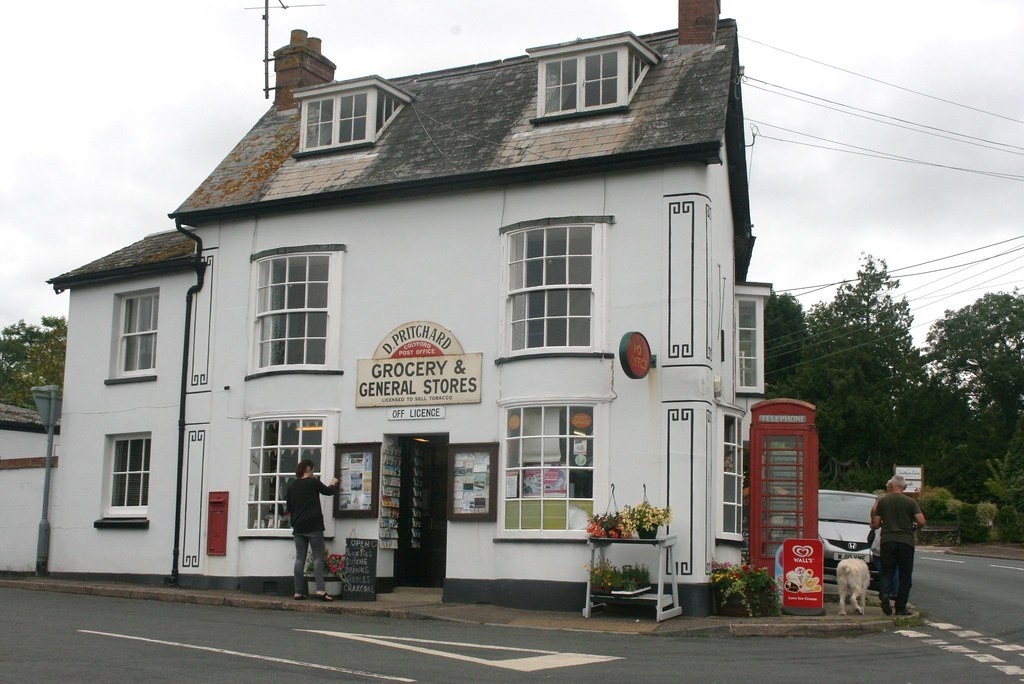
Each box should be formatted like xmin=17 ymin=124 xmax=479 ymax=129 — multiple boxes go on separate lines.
xmin=871 ymin=527 xmax=881 ymax=556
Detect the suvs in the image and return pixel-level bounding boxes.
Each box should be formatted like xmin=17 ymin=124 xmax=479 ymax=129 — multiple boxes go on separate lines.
xmin=817 ymin=489 xmax=886 ymax=589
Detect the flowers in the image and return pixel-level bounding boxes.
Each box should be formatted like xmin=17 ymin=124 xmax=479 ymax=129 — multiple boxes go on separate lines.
xmin=630 ymin=499 xmax=668 ymax=531
xmin=711 ymin=559 xmax=781 ymax=618
xmin=584 ymin=555 xmax=616 ymax=587
xmin=303 ymin=548 xmax=346 ymax=579
xmin=587 ymin=513 xmax=627 ymax=538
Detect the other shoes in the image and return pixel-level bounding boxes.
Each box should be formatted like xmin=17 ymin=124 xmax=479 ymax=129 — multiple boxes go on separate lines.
xmin=890 ymin=595 xmax=897 ymax=600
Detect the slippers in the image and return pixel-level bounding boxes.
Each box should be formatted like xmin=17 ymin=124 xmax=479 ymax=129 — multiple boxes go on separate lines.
xmin=315 ymin=591 xmax=333 ymax=601
xmin=294 ymin=593 xmax=308 ymax=600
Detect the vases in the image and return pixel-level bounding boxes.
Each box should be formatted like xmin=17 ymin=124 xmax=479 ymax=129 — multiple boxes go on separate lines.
xmin=308 ymin=577 xmax=342 ymax=600
xmin=635 ymin=524 xmax=658 ymax=539
xmin=714 ymin=582 xmax=771 ymax=617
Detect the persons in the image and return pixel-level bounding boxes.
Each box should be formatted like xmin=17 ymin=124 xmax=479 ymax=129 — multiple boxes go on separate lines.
xmin=285 ymin=460 xmax=339 ymax=602
xmin=870 ymin=475 xmax=925 ymax=616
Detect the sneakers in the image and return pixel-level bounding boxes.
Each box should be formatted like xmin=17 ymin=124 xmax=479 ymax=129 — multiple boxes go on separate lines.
xmin=881 ymin=601 xmax=893 ymax=616
xmin=897 ymin=610 xmax=912 ymax=615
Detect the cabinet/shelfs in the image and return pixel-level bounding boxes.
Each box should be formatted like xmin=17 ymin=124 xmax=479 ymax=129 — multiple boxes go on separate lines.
xmin=582 ymin=534 xmax=682 ymax=622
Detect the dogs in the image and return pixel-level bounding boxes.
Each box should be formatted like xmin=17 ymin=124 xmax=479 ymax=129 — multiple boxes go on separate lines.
xmin=836 ymin=558 xmax=871 ymax=616
